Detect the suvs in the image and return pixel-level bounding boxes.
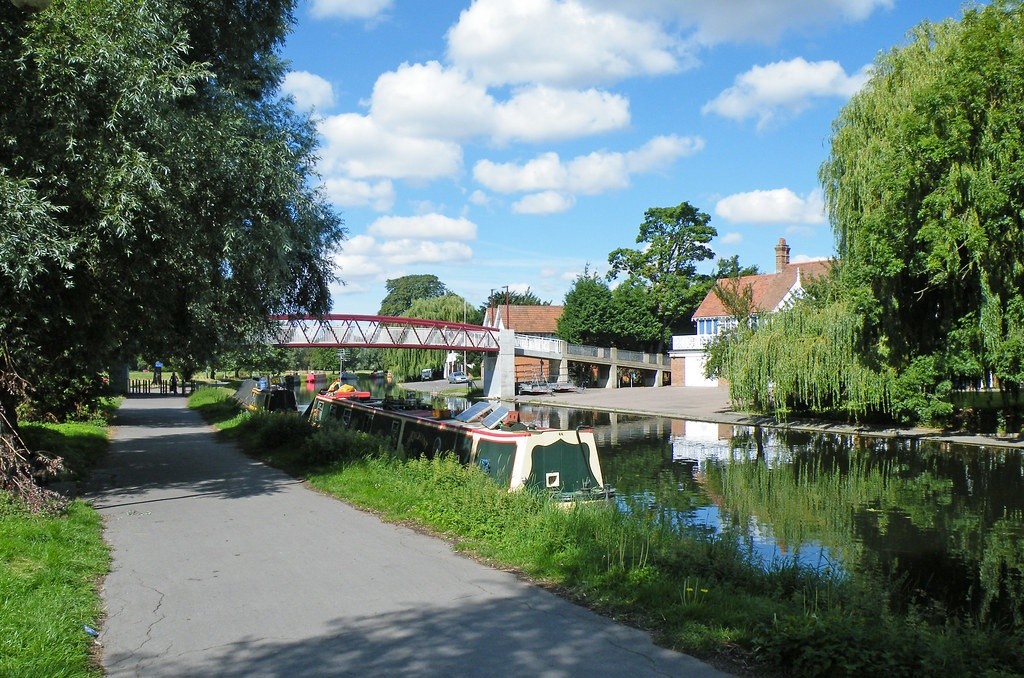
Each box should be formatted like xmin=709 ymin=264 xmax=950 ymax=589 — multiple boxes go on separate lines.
xmin=421 ymin=368 xmax=433 ymax=382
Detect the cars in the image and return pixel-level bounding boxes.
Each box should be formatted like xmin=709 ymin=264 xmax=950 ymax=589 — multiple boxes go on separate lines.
xmin=448 ymin=371 xmax=471 ymax=384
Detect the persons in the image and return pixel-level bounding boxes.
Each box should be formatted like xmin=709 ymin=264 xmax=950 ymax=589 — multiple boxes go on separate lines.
xmin=170 ymin=372 xmax=179 ymax=395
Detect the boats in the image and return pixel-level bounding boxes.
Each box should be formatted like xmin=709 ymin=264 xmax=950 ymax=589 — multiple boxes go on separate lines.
xmin=305 ymin=372 xmax=328 ymax=383
xmin=307 ymin=387 xmax=618 ymax=519
xmin=339 ymin=372 xmax=357 ymax=380
xmin=232 ymin=378 xmax=298 ymax=414
